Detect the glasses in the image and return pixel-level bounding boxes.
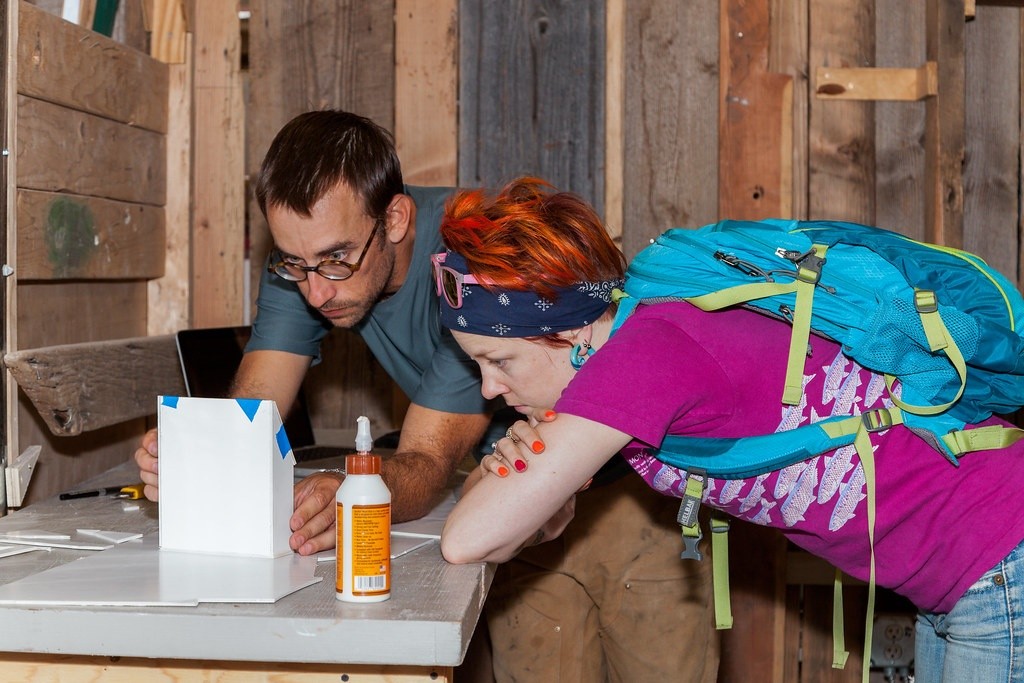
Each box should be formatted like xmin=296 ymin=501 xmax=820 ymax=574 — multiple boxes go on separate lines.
xmin=429 ymin=253 xmax=557 ymax=308
xmin=267 ymin=215 xmax=382 ymax=281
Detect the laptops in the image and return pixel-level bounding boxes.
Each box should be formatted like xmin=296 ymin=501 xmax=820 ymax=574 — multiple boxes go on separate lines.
xmin=175 ymin=325 xmax=400 ymax=477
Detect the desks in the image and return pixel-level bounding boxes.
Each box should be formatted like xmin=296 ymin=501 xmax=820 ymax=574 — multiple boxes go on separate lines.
xmin=0 ymin=458 xmax=498 ymax=683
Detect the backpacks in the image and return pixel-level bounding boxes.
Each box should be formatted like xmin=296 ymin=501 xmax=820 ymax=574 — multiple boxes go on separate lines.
xmin=608 ymin=219 xmax=1024 ymax=480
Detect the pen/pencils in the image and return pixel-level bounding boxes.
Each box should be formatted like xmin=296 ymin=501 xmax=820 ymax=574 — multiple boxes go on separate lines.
xmin=59 ymin=485 xmax=129 ymax=500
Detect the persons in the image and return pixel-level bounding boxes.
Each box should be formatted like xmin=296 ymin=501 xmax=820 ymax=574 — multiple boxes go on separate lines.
xmin=136 ymin=108 xmax=720 ymax=680
xmin=429 ymin=175 xmax=1024 ymax=683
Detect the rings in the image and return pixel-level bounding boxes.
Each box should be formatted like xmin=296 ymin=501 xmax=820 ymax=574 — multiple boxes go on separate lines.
xmin=490 ymin=437 xmax=507 ymax=454
xmin=505 ymin=424 xmax=518 ymax=443
